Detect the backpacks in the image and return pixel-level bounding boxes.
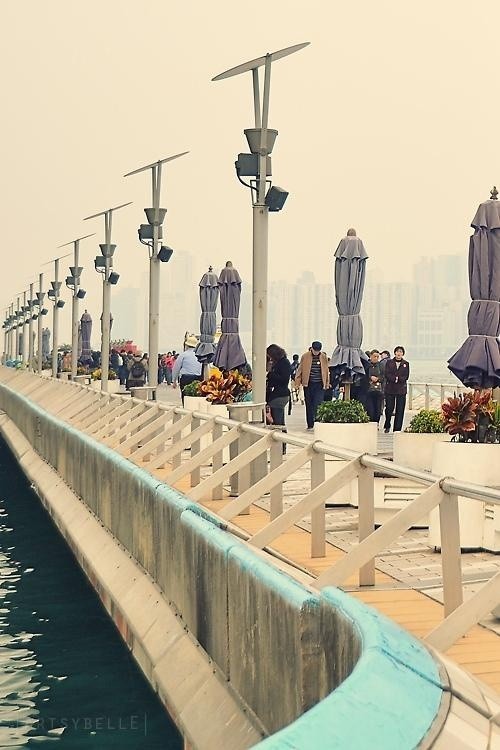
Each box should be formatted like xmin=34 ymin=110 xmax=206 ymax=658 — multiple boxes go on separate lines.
xmin=131 ymin=357 xmax=144 ymax=378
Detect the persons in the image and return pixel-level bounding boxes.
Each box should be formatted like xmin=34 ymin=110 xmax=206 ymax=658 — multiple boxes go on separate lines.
xmin=112 ymin=342 xmax=411 ymax=458
xmin=58 ymin=350 xmax=99 ymax=372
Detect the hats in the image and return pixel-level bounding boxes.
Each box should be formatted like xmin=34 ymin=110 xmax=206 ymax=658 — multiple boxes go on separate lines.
xmin=185 ymin=337 xmax=198 ymax=347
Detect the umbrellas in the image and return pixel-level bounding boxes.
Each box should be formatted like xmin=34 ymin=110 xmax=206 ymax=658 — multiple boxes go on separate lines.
xmin=80 ymin=310 xmax=92 ymax=366
xmin=212 ymin=262 xmax=249 ymax=370
xmin=448 ymin=186 xmax=500 ymax=393
xmin=99 ymin=311 xmax=113 ymax=364
xmin=328 ymin=230 xmax=372 ymax=400
xmin=194 ymin=266 xmax=219 ymax=382
xmin=18 ymin=326 xmax=50 ymax=357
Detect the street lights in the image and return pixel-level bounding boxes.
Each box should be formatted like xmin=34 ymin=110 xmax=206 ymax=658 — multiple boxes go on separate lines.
xmin=211 ymin=40 xmax=311 ymax=425
